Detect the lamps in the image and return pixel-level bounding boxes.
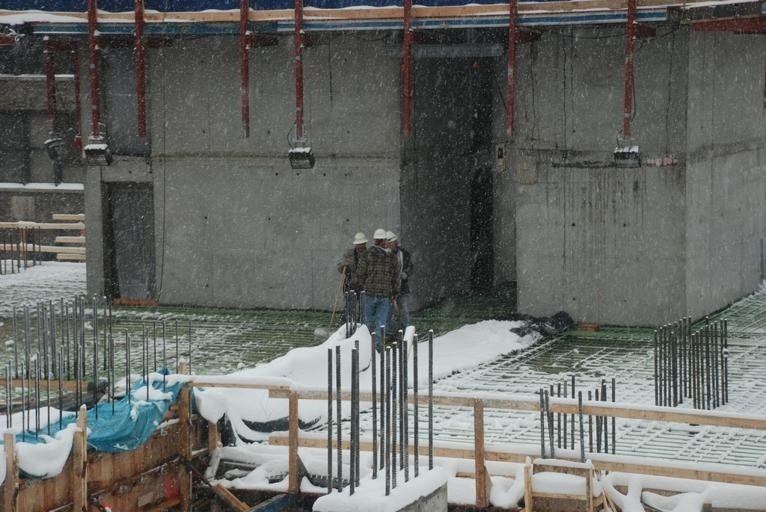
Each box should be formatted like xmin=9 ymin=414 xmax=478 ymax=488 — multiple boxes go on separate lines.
xmin=287 ymin=146 xmax=314 ymax=170
xmin=613 ymin=145 xmax=642 ymax=169
xmin=80 ymin=135 xmax=114 ymax=167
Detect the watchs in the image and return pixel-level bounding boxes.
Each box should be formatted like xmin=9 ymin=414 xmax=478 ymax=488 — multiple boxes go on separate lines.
xmin=354 ymin=228 xmax=401 ymax=351
xmin=336 ymin=233 xmax=369 ymax=329
xmin=384 ymin=231 xmax=413 ymax=341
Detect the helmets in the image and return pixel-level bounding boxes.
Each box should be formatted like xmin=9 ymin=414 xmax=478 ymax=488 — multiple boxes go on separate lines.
xmin=353 ymin=233 xmax=367 ymax=244
xmin=386 ymin=232 xmax=397 ymax=242
xmin=374 ymin=229 xmax=386 ymax=240
